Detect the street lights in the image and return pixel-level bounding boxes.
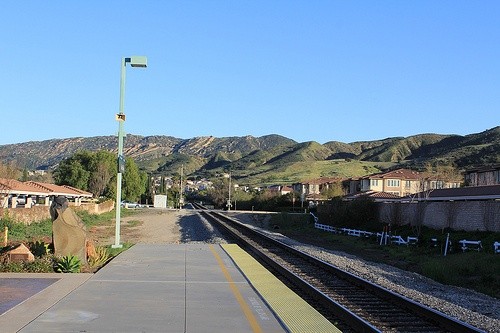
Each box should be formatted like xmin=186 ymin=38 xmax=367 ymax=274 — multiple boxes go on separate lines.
xmin=111 ymin=55 xmax=147 ymax=249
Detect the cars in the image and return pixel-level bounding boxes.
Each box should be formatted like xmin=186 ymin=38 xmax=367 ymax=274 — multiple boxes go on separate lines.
xmin=121 ymin=199 xmax=142 ymax=208
